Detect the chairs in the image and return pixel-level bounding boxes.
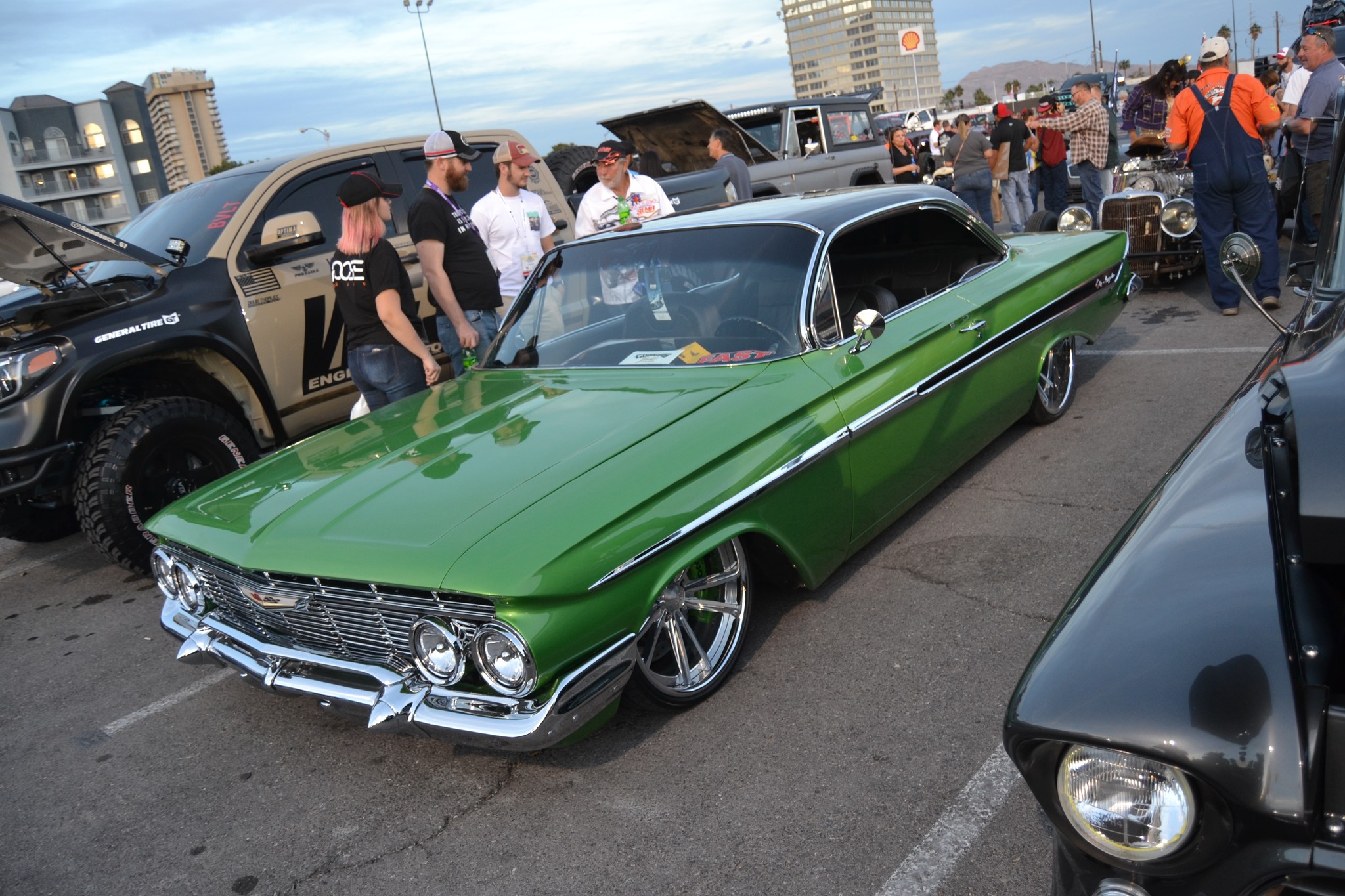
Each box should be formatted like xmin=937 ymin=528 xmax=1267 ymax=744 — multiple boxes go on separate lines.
xmin=836 ymin=284 xmax=898 ymax=339
xmin=623 ymin=291 xmax=721 ymax=338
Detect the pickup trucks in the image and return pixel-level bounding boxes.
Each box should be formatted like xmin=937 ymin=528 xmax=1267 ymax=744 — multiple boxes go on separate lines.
xmin=873 ymin=107 xmax=934 ymax=140
xmin=0 ymin=128 xmax=741 ymax=582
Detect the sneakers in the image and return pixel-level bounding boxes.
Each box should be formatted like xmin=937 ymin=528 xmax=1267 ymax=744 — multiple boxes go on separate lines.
xmin=1221 ymin=306 xmax=1240 ymax=316
xmin=1261 ymin=296 xmax=1281 ymax=309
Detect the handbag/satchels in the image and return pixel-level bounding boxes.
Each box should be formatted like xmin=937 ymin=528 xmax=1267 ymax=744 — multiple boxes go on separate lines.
xmin=1035 ymin=138 xmax=1044 ymax=162
xmin=991 ymin=141 xmax=1011 ymax=181
xmin=977 ymin=186 xmax=1003 ymax=223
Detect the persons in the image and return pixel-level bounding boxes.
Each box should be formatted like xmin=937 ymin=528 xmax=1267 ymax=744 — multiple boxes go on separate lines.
xmin=1165 ymin=36 xmax=1282 ymax=315
xmin=407 ymin=129 xmax=504 ymax=377
xmin=574 ymin=139 xmax=677 ymax=315
xmin=1258 ymin=47 xmax=1301 ymax=204
xmin=1011 ymin=111 xmax=1018 ymax=119
xmin=885 ymin=119 xmax=958 ymax=185
xmin=1025 ymin=81 xmax=1109 ymax=229
xmin=1285 ymin=24 xmax=1345 ymax=298
xmin=469 ymin=141 xmax=565 ymax=348
xmin=1091 ymin=84 xmax=1121 ymax=198
xmin=1185 ymin=69 xmax=1202 ymax=88
xmin=944 ymin=114 xmax=994 ymax=231
xmin=1120 ymin=52 xmax=1192 ymax=145
xmin=990 ymin=103 xmax=1034 ymax=233
xmin=1034 ymin=94 xmax=1070 ymax=217
xmin=331 ymin=172 xmax=442 ymax=411
xmin=1021 ymin=106 xmax=1041 ymax=215
xmin=707 ymin=128 xmax=753 ymax=202
xmin=1277 ymin=35 xmax=1314 ymax=240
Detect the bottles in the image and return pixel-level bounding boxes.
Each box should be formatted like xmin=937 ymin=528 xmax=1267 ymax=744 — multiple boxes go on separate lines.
xmin=618 ymin=196 xmax=634 ymax=225
xmin=462 ymin=346 xmax=479 ymax=372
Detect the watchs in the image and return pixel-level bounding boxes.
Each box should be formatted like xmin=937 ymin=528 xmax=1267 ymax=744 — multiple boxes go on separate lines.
xmin=555 ymin=280 xmax=565 ymax=286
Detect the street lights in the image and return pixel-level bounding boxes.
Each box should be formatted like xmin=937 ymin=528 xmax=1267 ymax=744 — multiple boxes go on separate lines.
xmin=403 ymin=0 xmax=444 ymax=135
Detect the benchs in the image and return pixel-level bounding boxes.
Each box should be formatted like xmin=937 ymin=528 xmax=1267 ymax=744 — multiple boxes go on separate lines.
xmin=753 ymin=244 xmax=979 ymax=332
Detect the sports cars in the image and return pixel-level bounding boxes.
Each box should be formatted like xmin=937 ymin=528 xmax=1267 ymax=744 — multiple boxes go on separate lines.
xmin=144 ymin=185 xmax=1137 ymax=752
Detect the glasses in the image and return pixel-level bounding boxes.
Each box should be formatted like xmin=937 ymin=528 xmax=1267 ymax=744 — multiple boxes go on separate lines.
xmin=1071 ymin=89 xmax=1081 ymax=98
xmin=1306 ymin=26 xmax=1329 ymax=46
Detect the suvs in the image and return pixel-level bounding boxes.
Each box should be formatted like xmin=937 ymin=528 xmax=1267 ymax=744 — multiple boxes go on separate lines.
xmin=565 ymin=95 xmax=894 ymax=232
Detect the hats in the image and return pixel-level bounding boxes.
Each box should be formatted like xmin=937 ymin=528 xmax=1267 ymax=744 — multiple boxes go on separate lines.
xmin=591 ymin=139 xmax=627 ymax=166
xmin=423 ymin=130 xmax=484 ymax=163
xmin=1272 ymin=47 xmax=1295 ymax=59
xmin=491 ymin=140 xmax=541 ymax=167
xmin=992 ymin=103 xmax=1013 ymax=118
xmin=896 ymin=123 xmax=907 ymax=129
xmin=1037 ymin=94 xmax=1053 ymax=113
xmin=621 ymin=141 xmax=640 ymax=156
xmin=335 ymin=171 xmax=403 ymax=209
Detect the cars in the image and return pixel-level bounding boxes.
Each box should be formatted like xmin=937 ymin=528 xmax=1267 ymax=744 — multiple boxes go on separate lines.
xmin=1003 ymin=74 xmax=1345 ymax=896
xmin=1023 ymin=124 xmax=1298 ymax=287
